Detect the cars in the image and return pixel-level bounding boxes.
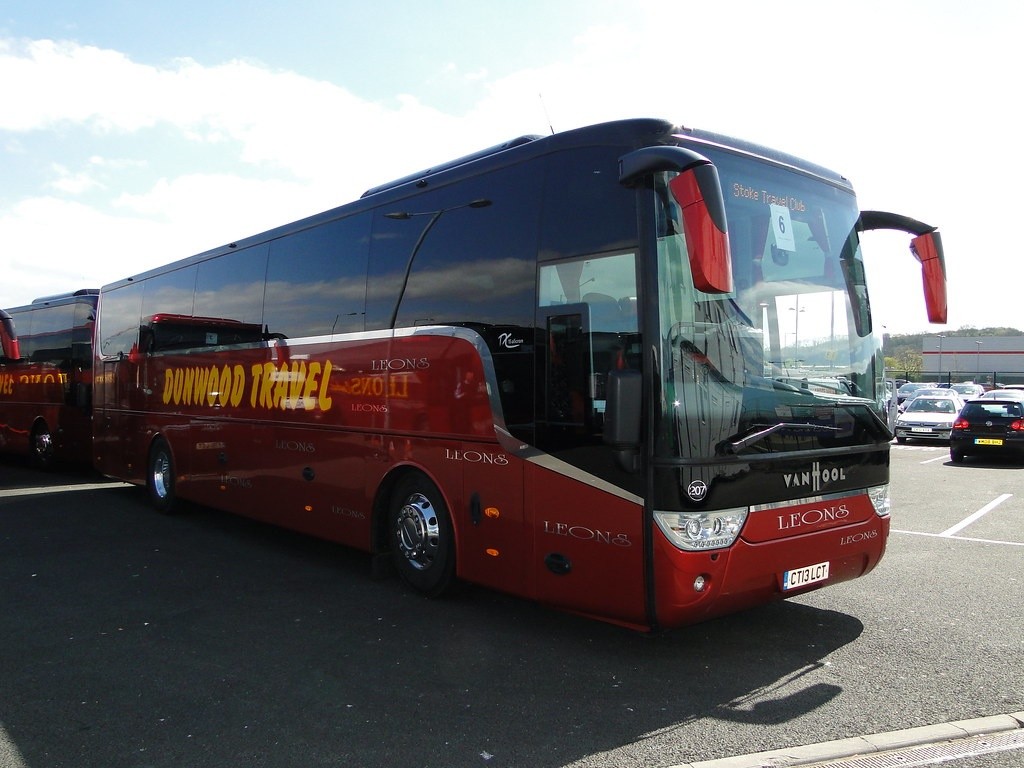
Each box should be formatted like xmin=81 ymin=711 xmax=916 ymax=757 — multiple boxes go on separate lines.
xmin=887 ymin=382 xmax=938 ymax=405
xmin=949 ymin=382 xmax=985 ymax=401
xmin=980 ymin=383 xmax=993 ymax=391
xmin=900 ymin=388 xmax=958 ymax=410
xmin=1003 ymin=384 xmax=1024 ymax=392
xmin=949 ymin=393 xmax=1024 ymax=465
xmin=837 ymin=378 xmax=1005 ymax=390
xmin=976 ymin=390 xmax=1024 ymax=412
xmin=894 ymin=395 xmax=966 ymax=444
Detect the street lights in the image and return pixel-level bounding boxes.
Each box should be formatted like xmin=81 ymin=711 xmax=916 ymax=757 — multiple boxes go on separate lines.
xmin=788 ymin=307 xmax=805 ymax=367
xmin=936 ymin=335 xmax=946 ymax=382
xmin=975 ymin=341 xmax=982 ymax=384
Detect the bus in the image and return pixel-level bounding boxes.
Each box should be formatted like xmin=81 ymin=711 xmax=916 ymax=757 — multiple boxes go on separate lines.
xmin=93 ymin=114 xmax=949 ymax=631
xmin=0 ymin=289 xmax=97 ymax=481
xmin=18 ymin=322 xmax=93 ymax=383
xmin=102 ymin=312 xmax=292 ymax=467
